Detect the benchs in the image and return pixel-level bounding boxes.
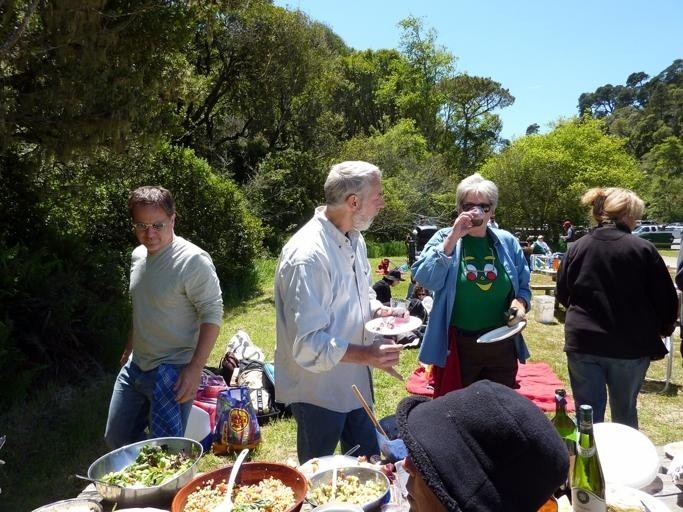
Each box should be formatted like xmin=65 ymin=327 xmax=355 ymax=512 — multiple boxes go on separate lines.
xmin=529 ymin=283 xmax=557 ymax=295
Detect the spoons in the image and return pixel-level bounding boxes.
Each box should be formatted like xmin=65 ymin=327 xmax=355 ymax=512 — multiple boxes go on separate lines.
xmin=213 ymin=449 xmax=248 ymax=512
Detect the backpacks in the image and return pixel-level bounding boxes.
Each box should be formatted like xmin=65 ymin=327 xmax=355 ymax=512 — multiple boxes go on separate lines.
xmin=235 ymin=359 xmax=279 ymax=420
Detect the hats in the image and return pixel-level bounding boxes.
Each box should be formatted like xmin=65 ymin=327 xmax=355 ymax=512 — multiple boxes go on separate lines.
xmin=384 ymin=269 xmax=405 ymax=282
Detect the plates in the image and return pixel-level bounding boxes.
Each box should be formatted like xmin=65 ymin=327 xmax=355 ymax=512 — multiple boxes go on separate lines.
xmin=364 ymin=316 xmax=422 ymax=334
xmin=304 ymin=467 xmax=390 ymax=510
xmin=574 ymin=423 xmax=659 ymax=490
xmin=476 ymin=322 xmax=526 ymax=343
xmin=604 ymin=485 xmax=670 ymax=512
xmin=298 ymin=456 xmax=358 ymax=475
xmin=32 ymin=498 xmax=103 ymax=511
xmin=665 ymin=441 xmax=682 ymax=454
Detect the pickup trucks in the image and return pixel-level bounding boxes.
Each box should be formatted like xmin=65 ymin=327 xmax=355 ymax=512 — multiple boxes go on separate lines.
xmin=631 ymin=224 xmax=680 ymax=242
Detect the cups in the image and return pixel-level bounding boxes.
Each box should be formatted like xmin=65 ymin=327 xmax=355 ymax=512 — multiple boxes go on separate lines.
xmin=470 ymin=206 xmax=483 ymax=227
xmin=390 ymin=298 xmax=411 ymax=320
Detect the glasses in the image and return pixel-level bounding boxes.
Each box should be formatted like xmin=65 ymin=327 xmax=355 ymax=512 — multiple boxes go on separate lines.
xmin=131 ymin=221 xmax=166 ymax=231
xmin=461 ymin=246 xmax=494 ymax=273
xmin=460 ymin=201 xmax=492 ymax=213
xmin=417 ymin=293 xmax=426 ymax=296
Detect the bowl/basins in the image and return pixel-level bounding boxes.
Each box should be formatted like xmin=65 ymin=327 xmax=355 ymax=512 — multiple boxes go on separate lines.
xmin=375 ymin=415 xmax=410 ymax=461
xmin=172 ymin=463 xmax=308 ymax=512
xmin=144 ymin=405 xmax=212 ymax=455
xmin=87 ymin=436 xmax=203 ymax=506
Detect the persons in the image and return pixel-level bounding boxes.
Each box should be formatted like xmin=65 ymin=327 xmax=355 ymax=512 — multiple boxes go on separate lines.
xmin=554 ymin=187 xmax=680 ymax=431
xmin=526 ymin=235 xmax=546 ymax=255
xmin=674 ymin=238 xmax=683 ymax=359
xmin=372 ymin=270 xmax=405 ymax=307
xmin=376 ymin=258 xmax=391 ymax=275
xmin=537 ymin=235 xmax=551 ymax=252
xmin=102 ymin=186 xmax=224 ymax=453
xmin=411 ymin=173 xmax=532 ymax=401
xmin=413 ymin=282 xmax=430 ymax=301
xmin=561 ymin=220 xmax=574 ymax=249
xmin=273 ymin=160 xmax=409 ymax=467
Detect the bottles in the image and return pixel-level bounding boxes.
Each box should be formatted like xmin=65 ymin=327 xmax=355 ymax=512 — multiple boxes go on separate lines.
xmin=552 ymin=389 xmax=577 ymax=489
xmin=573 ymin=405 xmax=607 ymax=512
